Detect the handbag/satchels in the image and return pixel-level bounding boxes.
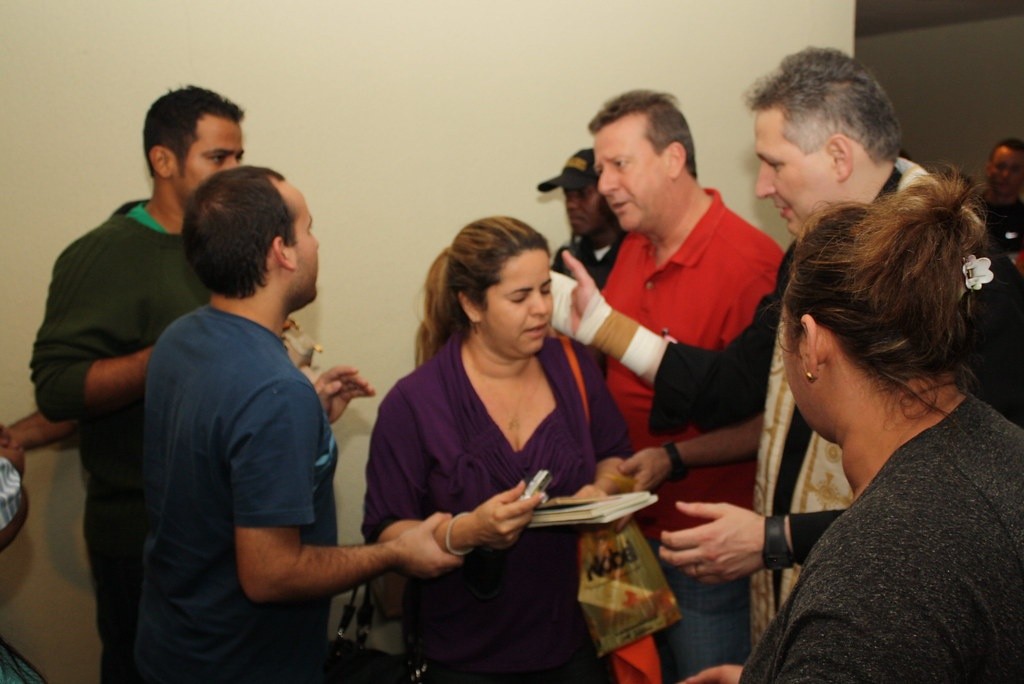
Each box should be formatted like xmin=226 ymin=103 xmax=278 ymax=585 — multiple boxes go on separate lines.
xmin=321 ymin=514 xmax=431 ymax=684
xmin=576 ymin=473 xmax=682 ymax=658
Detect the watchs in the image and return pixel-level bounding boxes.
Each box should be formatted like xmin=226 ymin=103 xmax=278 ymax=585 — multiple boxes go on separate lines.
xmin=664 ymin=443 xmax=687 ymax=480
xmin=763 ymin=516 xmax=794 ymax=570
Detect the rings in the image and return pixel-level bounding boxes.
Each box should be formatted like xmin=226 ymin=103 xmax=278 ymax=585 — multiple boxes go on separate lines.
xmin=695 ymin=566 xmax=700 ymax=575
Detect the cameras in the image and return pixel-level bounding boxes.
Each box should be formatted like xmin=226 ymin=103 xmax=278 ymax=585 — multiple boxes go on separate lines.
xmin=519 ymin=469 xmax=552 ymax=504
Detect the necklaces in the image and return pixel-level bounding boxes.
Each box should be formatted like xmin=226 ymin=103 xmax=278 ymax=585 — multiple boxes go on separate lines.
xmin=501 ymin=402 xmax=521 ymax=430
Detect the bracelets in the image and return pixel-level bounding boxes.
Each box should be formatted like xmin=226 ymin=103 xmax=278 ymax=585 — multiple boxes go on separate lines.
xmin=445 ymin=512 xmax=474 ymax=556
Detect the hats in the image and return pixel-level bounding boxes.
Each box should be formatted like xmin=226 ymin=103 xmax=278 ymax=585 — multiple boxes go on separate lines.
xmin=538 ymin=149 xmax=598 ymax=192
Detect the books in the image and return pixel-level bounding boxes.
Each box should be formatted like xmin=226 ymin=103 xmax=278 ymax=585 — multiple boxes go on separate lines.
xmin=527 ymin=492 xmax=658 ymax=527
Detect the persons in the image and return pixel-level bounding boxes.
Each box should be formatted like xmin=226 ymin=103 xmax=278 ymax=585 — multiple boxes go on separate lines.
xmin=133 ymin=166 xmax=463 ymax=684
xmin=358 ymin=216 xmax=635 ymax=684
xmin=28 ymin=85 xmax=244 ymax=684
xmin=537 ymin=149 xmax=627 ymax=294
xmin=684 ymin=175 xmax=1024 ymax=684
xmin=971 ymin=139 xmax=1024 ymax=254
xmin=549 ymin=46 xmax=1024 ymax=651
xmin=593 ymin=89 xmax=784 ymax=684
xmin=0 ymin=410 xmax=81 ymax=683
